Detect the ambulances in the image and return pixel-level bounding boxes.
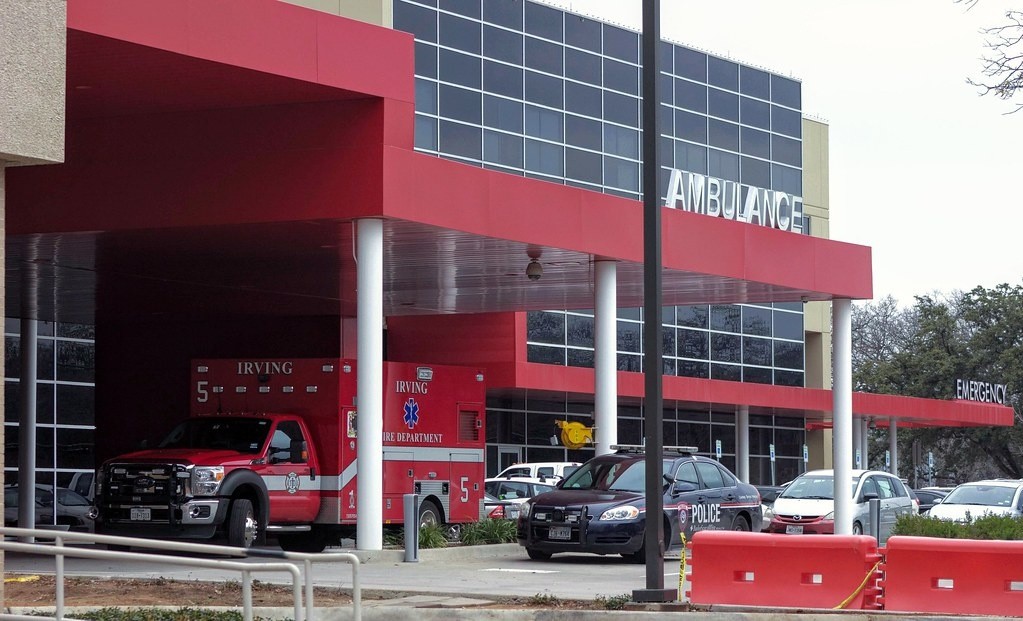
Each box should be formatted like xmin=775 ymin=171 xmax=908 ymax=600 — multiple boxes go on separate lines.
xmin=84 ymin=353 xmax=488 ymax=559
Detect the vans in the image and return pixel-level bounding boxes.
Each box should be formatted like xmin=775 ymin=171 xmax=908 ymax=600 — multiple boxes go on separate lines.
xmin=69 ymin=471 xmax=98 ymax=504
xmin=493 ymin=461 xmax=593 ymax=490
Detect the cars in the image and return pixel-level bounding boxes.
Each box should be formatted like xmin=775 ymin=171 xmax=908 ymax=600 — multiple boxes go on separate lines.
xmin=747 ymin=477 xmax=957 ymax=531
xmin=759 ymin=469 xmax=913 ymax=547
xmin=440 ymin=475 xmax=593 ymax=542
xmin=3 ymin=483 xmax=97 ymax=541
xmin=920 ymin=477 xmax=1023 ymax=526
xmin=517 ymin=446 xmax=764 ymax=565
